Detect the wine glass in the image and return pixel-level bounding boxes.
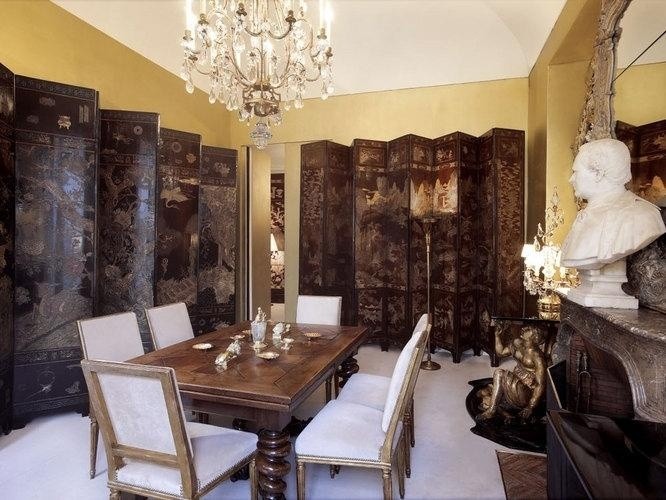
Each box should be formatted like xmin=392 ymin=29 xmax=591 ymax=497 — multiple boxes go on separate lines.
xmin=249 ymin=320 xmax=268 ymax=347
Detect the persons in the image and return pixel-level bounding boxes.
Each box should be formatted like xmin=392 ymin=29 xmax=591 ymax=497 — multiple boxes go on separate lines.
xmin=474 ymin=320 xmax=548 ymax=427
xmin=561 ymin=139 xmax=665 ymax=269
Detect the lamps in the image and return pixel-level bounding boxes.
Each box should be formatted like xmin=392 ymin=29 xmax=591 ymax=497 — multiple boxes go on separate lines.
xmin=520 ymin=184 xmax=581 ymax=320
xmin=413 ymin=216 xmax=443 ymax=371
xmin=178 ymin=0 xmax=336 ymax=151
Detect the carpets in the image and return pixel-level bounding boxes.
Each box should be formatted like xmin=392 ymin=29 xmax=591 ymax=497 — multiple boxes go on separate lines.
xmin=496 ymin=452 xmax=548 ymax=500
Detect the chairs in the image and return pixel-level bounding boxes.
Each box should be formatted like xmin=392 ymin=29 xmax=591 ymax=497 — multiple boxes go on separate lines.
xmin=295 ymin=295 xmax=342 ymax=404
xmin=294 ymin=329 xmax=428 ymax=500
xmin=144 ymin=302 xmax=195 ymax=351
xmin=80 ymin=358 xmax=260 ymax=500
xmin=334 ymin=313 xmax=433 ymax=478
xmin=76 ymin=311 xmax=145 ymax=480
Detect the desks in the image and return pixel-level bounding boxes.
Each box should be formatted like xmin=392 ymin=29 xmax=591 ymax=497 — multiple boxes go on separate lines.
xmin=546 ymin=408 xmax=666 ymax=500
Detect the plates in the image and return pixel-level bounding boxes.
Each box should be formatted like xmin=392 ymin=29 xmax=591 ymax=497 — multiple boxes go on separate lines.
xmin=302 ymin=332 xmax=322 ymax=338
xmin=230 ymin=330 xmax=252 ymax=340
xmin=256 ymin=351 xmax=280 ymax=360
xmin=192 ymin=342 xmax=215 ymax=350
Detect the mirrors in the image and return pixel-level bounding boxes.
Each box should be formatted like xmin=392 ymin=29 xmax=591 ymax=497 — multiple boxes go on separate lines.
xmin=569 ymin=0 xmax=666 ymax=315
